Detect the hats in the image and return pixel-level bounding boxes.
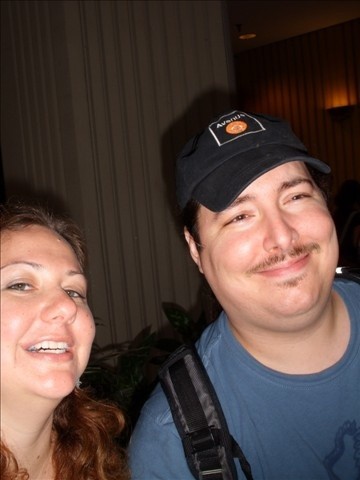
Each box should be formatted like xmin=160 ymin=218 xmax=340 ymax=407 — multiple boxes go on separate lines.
xmin=172 ymin=109 xmax=331 ymax=223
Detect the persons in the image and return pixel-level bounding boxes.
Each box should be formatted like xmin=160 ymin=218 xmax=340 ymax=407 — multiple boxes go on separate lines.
xmin=0 ymin=202 xmax=131 ymax=480
xmin=124 ymin=109 xmax=360 ymax=480
